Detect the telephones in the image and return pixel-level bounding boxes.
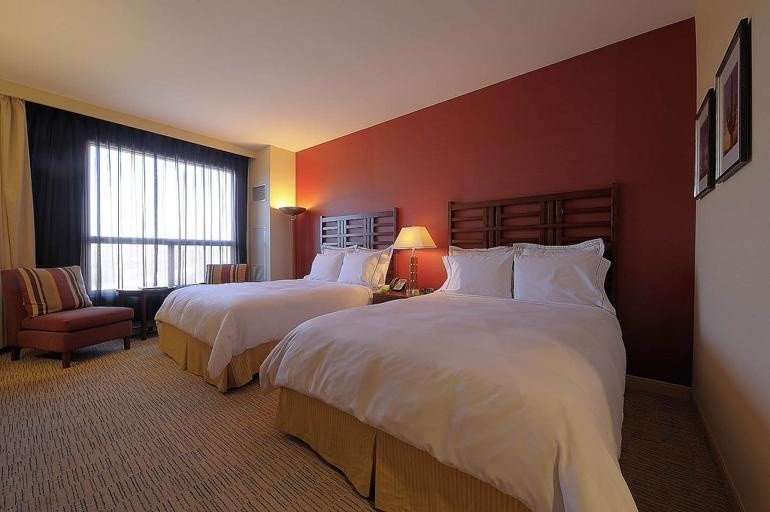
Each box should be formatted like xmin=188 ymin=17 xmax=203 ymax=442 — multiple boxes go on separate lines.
xmin=388 ymin=277 xmax=408 ymax=291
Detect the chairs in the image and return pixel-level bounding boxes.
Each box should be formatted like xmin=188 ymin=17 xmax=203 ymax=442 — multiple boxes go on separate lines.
xmin=0 ymin=268 xmax=134 ymax=368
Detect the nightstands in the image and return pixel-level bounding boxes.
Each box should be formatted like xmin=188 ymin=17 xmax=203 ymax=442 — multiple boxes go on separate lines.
xmin=373 ymin=290 xmax=425 ymax=304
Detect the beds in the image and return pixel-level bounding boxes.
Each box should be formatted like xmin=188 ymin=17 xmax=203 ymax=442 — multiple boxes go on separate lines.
xmin=274 ymin=181 xmax=640 ymax=512
xmin=160 ymin=207 xmax=399 ymax=394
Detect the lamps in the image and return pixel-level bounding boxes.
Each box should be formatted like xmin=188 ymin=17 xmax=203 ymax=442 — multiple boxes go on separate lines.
xmin=278 ymin=207 xmax=306 ymax=224
xmin=392 ymin=225 xmax=438 ymax=293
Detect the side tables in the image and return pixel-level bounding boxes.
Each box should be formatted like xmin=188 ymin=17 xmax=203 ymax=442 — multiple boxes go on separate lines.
xmin=116 ymin=289 xmax=168 ymax=341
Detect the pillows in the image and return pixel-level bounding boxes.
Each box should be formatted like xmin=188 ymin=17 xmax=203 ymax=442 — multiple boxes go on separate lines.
xmin=440 ymin=238 xmax=616 ymax=315
xmin=17 ymin=265 xmax=92 ymax=318
xmin=303 ymin=244 xmax=393 ymax=288
xmin=206 ymin=264 xmax=250 ymax=284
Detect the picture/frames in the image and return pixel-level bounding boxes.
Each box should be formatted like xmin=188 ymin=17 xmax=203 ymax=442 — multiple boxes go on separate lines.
xmin=713 ymin=17 xmax=751 ymax=183
xmin=693 ymin=88 xmax=715 ymax=200
